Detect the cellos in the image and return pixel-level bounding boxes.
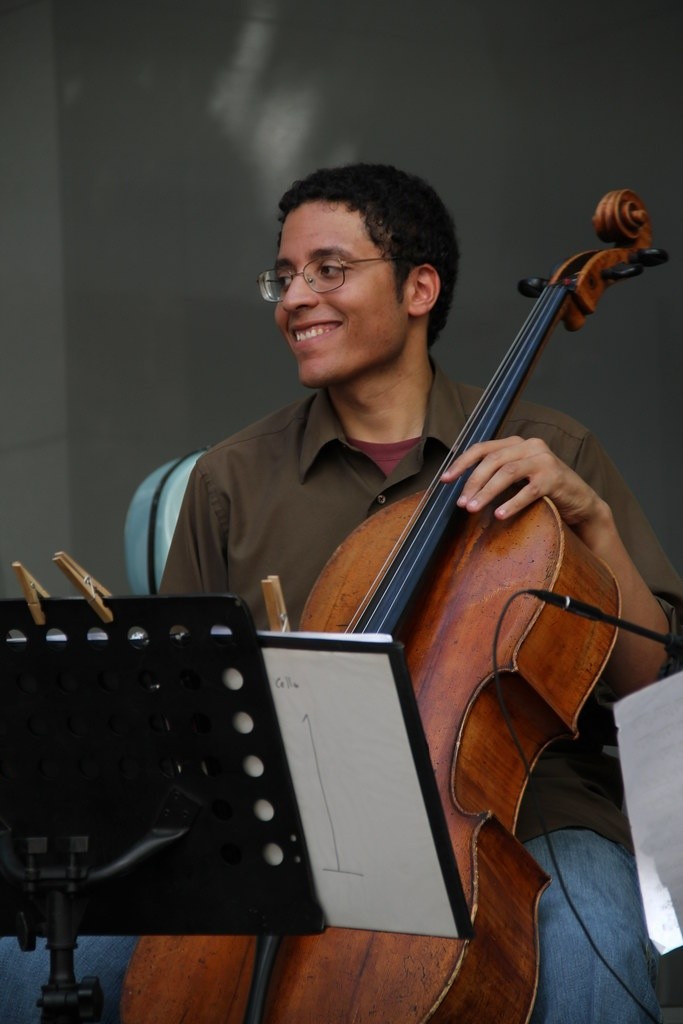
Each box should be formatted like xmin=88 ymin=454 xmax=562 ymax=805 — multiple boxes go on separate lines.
xmin=120 ymin=188 xmax=672 ymax=1022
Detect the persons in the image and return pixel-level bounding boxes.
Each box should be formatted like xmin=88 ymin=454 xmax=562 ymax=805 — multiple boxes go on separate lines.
xmin=1 ymin=164 xmax=683 ymax=1023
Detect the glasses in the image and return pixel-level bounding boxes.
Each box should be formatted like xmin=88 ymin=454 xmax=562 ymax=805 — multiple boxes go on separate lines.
xmin=255 ymin=255 xmax=396 ymax=303
